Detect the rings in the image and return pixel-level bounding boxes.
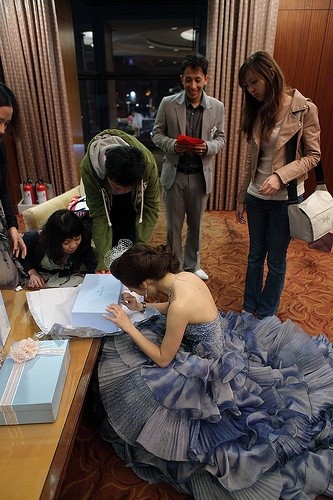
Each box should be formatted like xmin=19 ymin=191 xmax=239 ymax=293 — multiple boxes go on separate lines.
xmin=201 ymin=149 xmax=202 ymax=152
xmin=115 ymin=313 xmax=117 ymax=316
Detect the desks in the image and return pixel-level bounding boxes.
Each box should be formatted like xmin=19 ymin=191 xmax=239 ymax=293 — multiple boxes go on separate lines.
xmin=0 ymin=285 xmax=101 ymax=500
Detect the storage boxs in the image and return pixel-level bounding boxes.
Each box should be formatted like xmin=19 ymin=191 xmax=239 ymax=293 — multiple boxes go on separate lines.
xmin=0 ymin=339 xmax=70 ymax=425
xmin=71 ymin=274 xmax=122 ymax=332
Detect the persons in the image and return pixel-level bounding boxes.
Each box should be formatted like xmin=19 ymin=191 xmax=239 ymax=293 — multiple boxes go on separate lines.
xmin=98 ymin=242 xmax=333 ymax=500
xmin=19 ymin=209 xmax=97 ymax=288
xmin=78 ymin=128 xmax=160 ymax=274
xmin=0 ymin=83 xmax=27 ymax=286
xmin=151 ymin=52 xmax=225 ymax=280
xmin=235 ymin=50 xmax=321 ymax=320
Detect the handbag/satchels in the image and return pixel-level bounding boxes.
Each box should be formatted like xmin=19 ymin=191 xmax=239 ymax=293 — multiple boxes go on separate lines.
xmin=286 ymin=98 xmax=333 ymax=242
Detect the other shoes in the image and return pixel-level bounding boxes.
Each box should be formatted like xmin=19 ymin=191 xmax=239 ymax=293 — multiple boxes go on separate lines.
xmin=193 ymin=268 xmax=209 ymax=280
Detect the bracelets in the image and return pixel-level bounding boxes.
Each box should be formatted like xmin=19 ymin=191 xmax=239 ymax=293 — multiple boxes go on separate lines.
xmin=140 ymin=302 xmax=146 ymax=314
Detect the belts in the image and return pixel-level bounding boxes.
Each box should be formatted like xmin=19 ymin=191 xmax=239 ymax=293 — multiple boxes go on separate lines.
xmin=177 ymin=168 xmax=202 ymax=175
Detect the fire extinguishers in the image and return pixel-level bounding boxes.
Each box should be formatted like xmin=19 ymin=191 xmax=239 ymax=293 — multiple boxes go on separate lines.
xmin=34 ymin=177 xmax=48 ymax=204
xmin=23 ymin=177 xmax=35 ymax=205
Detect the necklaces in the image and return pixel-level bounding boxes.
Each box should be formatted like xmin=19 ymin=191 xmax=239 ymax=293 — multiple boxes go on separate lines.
xmin=168 ymin=274 xmax=178 ymax=305
